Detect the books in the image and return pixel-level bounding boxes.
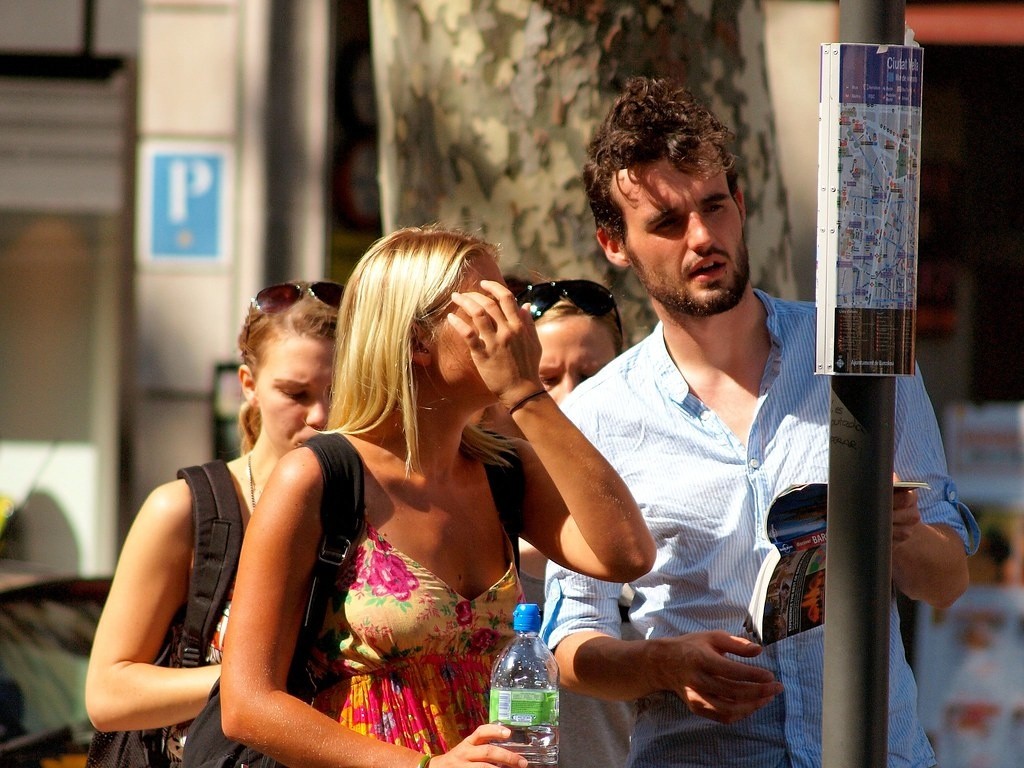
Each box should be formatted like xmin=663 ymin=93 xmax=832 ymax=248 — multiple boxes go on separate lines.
xmin=743 ymin=482 xmax=928 ymax=648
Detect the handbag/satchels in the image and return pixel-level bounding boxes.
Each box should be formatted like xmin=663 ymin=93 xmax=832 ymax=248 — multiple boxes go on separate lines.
xmin=85 ymin=459 xmax=244 ymax=768
xmin=181 ymin=434 xmax=367 ymax=767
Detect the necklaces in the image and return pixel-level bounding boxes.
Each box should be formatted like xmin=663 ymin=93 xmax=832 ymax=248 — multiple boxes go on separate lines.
xmin=247 ymin=453 xmax=256 ymax=507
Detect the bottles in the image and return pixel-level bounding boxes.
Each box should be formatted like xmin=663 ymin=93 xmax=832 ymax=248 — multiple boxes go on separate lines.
xmin=488 ymin=604 xmax=562 ymax=768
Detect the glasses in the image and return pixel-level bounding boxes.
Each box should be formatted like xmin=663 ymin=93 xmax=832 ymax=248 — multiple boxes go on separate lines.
xmin=241 ymin=280 xmax=344 ymax=363
xmin=517 ymin=278 xmax=625 ymax=349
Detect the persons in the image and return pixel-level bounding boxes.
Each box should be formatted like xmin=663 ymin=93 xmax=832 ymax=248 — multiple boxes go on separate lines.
xmin=84 ymin=224 xmax=643 ymax=767
xmin=540 ymin=77 xmax=978 ymax=767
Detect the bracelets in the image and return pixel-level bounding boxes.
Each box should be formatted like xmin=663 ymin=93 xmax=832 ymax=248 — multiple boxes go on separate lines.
xmin=509 ymin=390 xmax=547 ymax=414
